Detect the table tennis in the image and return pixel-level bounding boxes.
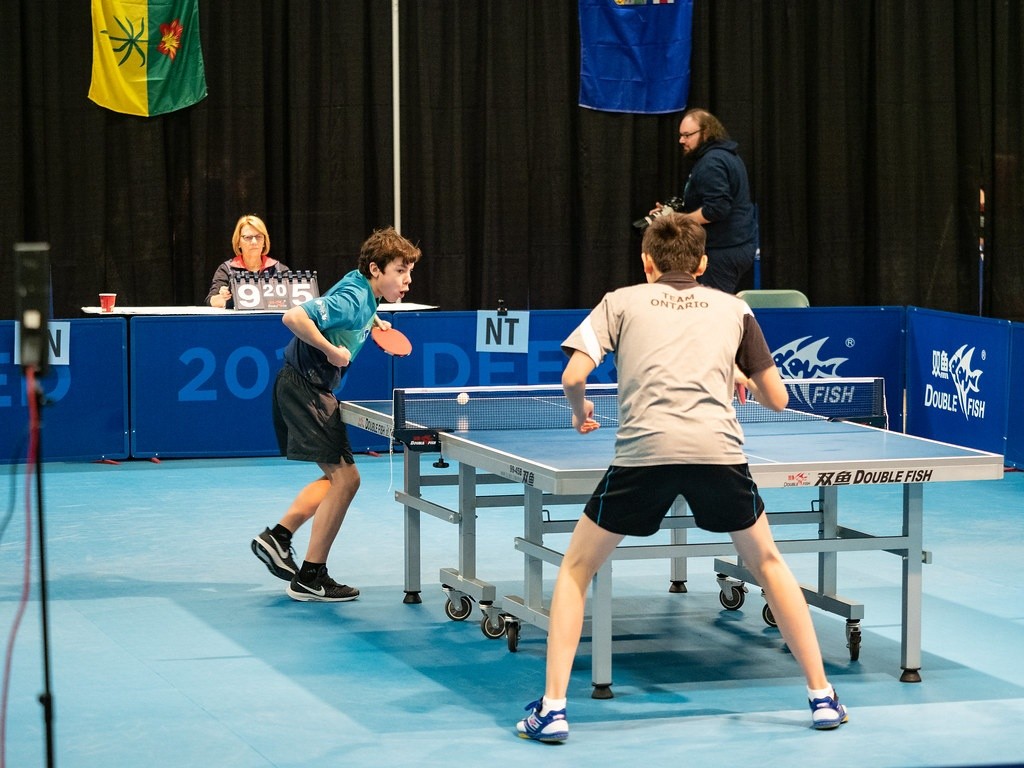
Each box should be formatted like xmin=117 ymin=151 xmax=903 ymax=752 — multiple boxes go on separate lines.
xmin=457 ymin=392 xmax=469 ymax=405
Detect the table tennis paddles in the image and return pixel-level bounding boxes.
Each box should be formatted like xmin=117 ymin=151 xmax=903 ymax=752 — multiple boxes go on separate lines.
xmin=735 ymin=381 xmax=749 ymax=409
xmin=370 ymin=317 xmax=413 ymax=358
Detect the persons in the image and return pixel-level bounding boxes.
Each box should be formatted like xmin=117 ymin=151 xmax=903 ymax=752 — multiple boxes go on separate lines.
xmin=514 ymin=213 xmax=850 ymax=743
xmin=203 ymin=215 xmax=291 ymax=309
xmin=649 ymin=107 xmax=758 ymax=298
xmin=251 ymin=224 xmax=422 ymax=603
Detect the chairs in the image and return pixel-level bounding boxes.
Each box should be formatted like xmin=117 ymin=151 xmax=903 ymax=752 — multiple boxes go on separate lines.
xmin=737 ymin=289 xmax=810 ymax=309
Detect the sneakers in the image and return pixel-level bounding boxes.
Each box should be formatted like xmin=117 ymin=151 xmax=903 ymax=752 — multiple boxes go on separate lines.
xmin=809 ymin=687 xmax=849 ymax=729
xmin=286 ymin=566 xmax=359 ymax=601
xmin=251 ymin=526 xmax=300 ymax=581
xmin=513 ymin=695 xmax=570 ymax=741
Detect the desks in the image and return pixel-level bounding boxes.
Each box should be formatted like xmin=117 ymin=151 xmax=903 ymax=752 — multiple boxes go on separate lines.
xmin=340 ymin=393 xmax=1003 ymax=701
xmin=83 ymin=301 xmax=440 ymax=319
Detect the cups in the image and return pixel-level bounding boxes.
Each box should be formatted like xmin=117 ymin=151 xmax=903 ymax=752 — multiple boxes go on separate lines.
xmin=99 ymin=293 xmax=117 ymax=313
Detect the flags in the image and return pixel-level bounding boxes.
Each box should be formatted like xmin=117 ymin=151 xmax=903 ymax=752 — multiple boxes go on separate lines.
xmin=87 ymin=0 xmax=209 ymax=117
xmin=577 ymin=0 xmax=694 ymax=113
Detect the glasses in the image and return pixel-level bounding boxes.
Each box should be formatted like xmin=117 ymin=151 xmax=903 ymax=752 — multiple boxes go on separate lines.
xmin=241 ymin=234 xmax=265 ymax=242
xmin=678 ymin=129 xmax=705 ymax=139
